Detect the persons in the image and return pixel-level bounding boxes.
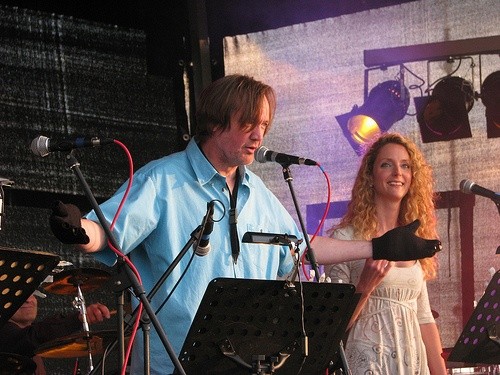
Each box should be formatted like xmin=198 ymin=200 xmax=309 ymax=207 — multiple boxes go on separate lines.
xmin=0 ymin=291 xmax=110 ymax=375
xmin=50 ymin=73 xmax=444 ymax=375
xmin=321 ymin=132 xmax=447 ymax=375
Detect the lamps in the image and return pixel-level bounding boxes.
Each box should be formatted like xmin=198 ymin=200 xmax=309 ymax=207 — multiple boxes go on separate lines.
xmin=414 ymin=56 xmax=476 ymax=144
xmin=336 ymin=62 xmax=410 ymax=157
xmin=474 ymin=52 xmax=500 ymax=139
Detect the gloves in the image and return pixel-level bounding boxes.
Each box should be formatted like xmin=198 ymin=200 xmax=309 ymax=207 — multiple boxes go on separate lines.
xmin=371 ymin=217 xmax=443 ymax=262
xmin=50 ymin=200 xmax=90 ymax=244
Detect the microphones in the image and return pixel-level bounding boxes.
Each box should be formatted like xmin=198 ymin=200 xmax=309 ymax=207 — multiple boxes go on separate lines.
xmin=254 ymin=146 xmax=317 ymax=168
xmin=193 ymin=202 xmax=214 ymax=257
xmin=30 ymin=135 xmax=114 ymax=157
xmin=460 ymin=179 xmax=500 ymax=205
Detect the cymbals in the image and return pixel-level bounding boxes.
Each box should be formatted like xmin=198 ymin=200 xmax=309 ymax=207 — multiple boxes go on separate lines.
xmin=38 ymin=265 xmax=111 ymax=295
xmin=32 ymin=334 xmax=103 ymax=359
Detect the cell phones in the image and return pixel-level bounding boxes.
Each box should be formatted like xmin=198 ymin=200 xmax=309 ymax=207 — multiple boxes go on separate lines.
xmin=242 ymin=232 xmax=297 ymax=245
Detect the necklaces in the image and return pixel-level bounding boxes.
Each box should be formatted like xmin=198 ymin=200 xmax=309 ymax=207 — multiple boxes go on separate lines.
xmin=199 ymin=144 xmax=242 ymax=265
